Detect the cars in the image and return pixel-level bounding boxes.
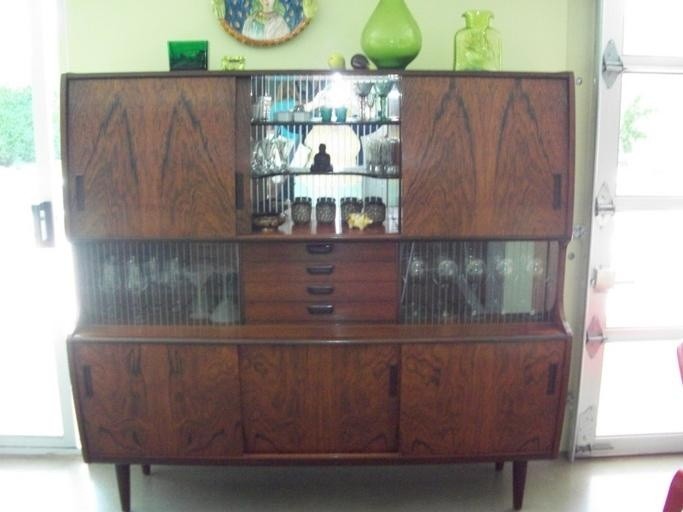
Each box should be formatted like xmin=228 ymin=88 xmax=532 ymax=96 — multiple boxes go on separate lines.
xmin=335 ymin=108 xmax=347 ymax=123
xmin=251 ymin=94 xmax=271 ymax=124
xmin=289 ymin=202 xmax=385 ymax=227
xmin=320 ymin=107 xmax=332 ymax=123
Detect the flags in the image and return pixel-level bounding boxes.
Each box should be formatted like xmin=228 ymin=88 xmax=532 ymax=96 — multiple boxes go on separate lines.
xmin=361 ymin=0 xmax=423 ymax=69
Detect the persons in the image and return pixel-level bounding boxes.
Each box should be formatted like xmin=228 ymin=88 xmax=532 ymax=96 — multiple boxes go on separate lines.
xmin=242 ymin=1 xmax=290 ymax=41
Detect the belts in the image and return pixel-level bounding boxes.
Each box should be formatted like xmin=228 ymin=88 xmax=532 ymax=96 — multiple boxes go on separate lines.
xmin=358 ymin=135 xmax=397 ymax=176
xmin=91 ymin=250 xmax=238 ymax=325
xmin=402 ymin=253 xmax=547 ymax=322
xmin=352 ymin=80 xmax=393 ymax=123
xmin=246 ymin=138 xmax=296 ymax=175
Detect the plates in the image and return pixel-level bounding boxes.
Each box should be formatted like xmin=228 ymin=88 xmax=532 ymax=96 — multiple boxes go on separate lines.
xmin=55 ymin=70 xmax=245 ymax=512
xmin=400 ymin=70 xmax=575 ymax=512
xmin=249 ymin=122 xmax=400 ymax=181
xmin=246 ymin=240 xmax=397 ymax=465
